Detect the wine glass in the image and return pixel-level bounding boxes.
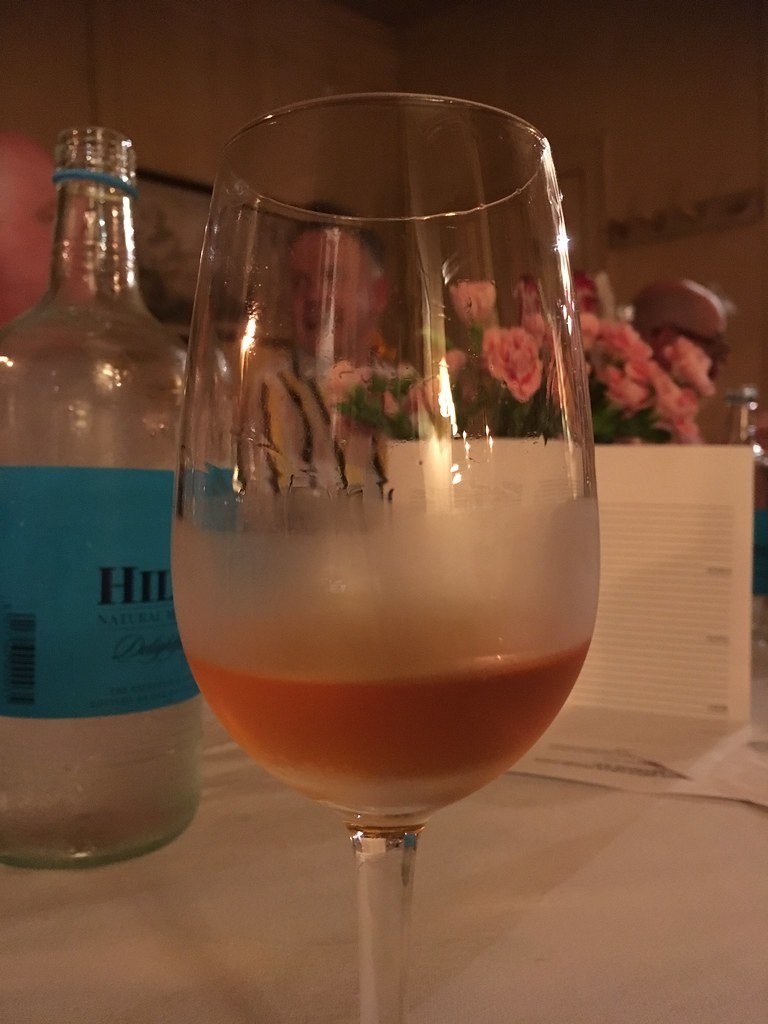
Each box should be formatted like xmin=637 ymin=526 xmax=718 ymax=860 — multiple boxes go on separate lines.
xmin=168 ymin=90 xmax=603 ymax=1024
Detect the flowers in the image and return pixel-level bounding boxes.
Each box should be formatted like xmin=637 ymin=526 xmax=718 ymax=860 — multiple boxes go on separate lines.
xmin=300 ymin=274 xmax=718 ymax=446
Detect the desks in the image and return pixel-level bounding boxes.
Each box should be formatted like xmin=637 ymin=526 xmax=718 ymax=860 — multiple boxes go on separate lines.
xmin=0 ymin=591 xmax=768 ymax=1024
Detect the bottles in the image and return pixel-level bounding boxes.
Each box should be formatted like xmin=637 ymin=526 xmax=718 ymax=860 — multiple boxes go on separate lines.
xmin=723 ymin=384 xmax=768 ymax=748
xmin=0 ymin=125 xmax=202 ymax=872
